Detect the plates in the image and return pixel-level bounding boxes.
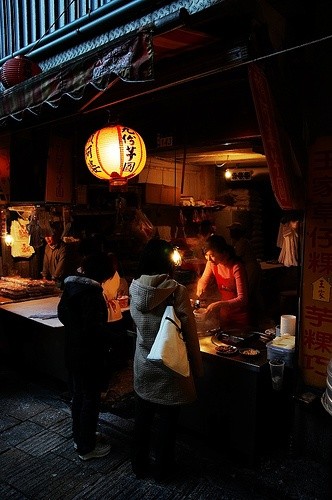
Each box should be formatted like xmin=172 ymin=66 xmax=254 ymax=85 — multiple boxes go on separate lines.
xmin=214 ymin=345 xmax=238 ymax=355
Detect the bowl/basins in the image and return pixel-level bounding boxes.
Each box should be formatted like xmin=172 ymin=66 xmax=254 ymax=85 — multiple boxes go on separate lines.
xmin=239 ymin=348 xmax=261 ymax=360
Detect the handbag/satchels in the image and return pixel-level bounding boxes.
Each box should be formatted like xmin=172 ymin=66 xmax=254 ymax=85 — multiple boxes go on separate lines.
xmin=147 ymin=289 xmax=191 ymax=378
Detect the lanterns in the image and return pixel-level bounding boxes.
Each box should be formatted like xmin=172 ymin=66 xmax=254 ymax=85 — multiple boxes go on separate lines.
xmin=0 ymin=53 xmax=43 ymax=89
xmin=84 ymin=124 xmax=148 ymax=192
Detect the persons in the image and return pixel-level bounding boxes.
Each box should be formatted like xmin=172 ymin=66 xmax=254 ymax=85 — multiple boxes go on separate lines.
xmin=58 ymin=252 xmax=111 ymax=461
xmin=195 ymin=234 xmax=249 ymax=330
xmin=128 ymin=240 xmax=202 ymax=484
xmin=240 ymin=212 xmax=266 ymax=269
xmin=40 ymin=227 xmax=67 ymax=291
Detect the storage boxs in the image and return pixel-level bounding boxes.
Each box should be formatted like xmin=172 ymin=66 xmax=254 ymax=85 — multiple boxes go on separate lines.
xmin=74 ymin=183 xmax=180 ymax=206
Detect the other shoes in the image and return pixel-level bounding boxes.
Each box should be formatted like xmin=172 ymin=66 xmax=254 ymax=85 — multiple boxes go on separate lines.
xmin=74 ymin=439 xmax=106 ymax=452
xmin=136 ymin=469 xmax=168 ymax=482
xmin=79 ymin=444 xmax=110 ymax=460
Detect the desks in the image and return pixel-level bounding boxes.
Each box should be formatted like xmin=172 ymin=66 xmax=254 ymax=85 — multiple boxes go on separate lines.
xmin=0 ymin=290 xmax=130 ymax=393
xmin=198 ymin=327 xmax=269 ymax=453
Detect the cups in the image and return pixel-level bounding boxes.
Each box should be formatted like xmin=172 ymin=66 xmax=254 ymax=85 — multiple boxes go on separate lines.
xmin=269 ymin=359 xmax=285 ymax=385
xmin=117 ymin=296 xmax=129 ymax=308
xmin=280 ymin=315 xmax=296 ymax=336
xmin=192 ymin=308 xmax=208 ymax=324
